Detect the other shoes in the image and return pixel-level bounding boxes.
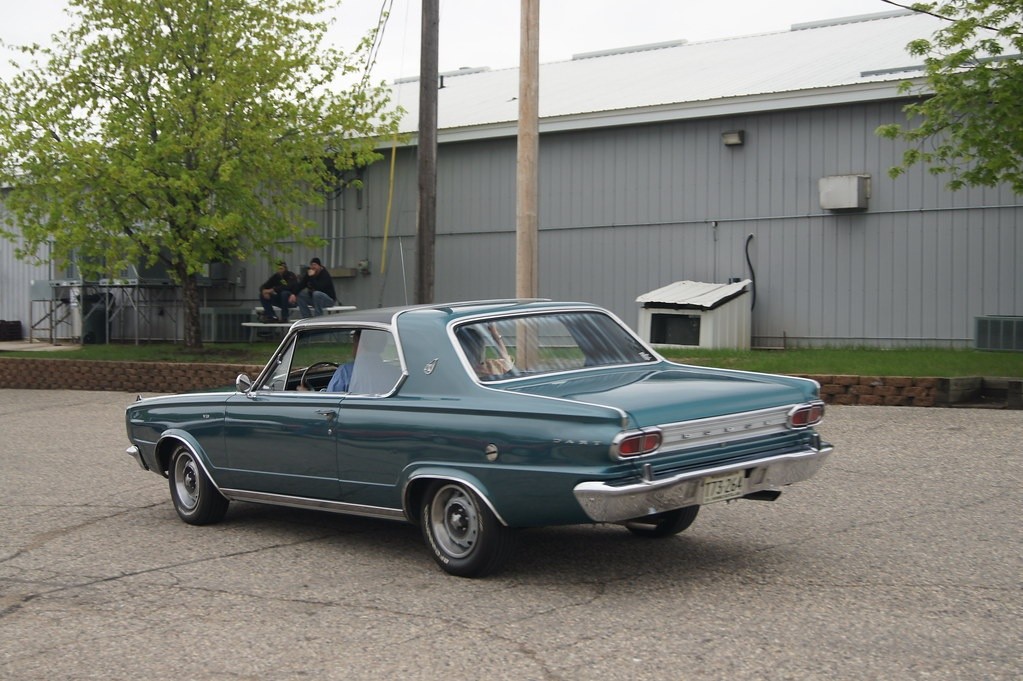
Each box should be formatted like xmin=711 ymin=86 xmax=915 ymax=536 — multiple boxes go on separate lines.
xmin=262 ymin=316 xmax=278 ymax=322
xmin=279 ymin=318 xmax=288 ymax=323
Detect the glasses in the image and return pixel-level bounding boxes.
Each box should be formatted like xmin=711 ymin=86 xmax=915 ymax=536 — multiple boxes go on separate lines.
xmin=350 ymin=331 xmax=356 ymax=337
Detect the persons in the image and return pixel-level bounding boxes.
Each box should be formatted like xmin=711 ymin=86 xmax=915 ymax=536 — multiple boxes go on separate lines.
xmin=259 ymin=263 xmax=298 ymax=324
xmin=456 ymin=322 xmax=514 ymax=378
xmin=296 ymin=328 xmax=394 ymax=394
xmin=288 ymin=257 xmax=336 ymax=318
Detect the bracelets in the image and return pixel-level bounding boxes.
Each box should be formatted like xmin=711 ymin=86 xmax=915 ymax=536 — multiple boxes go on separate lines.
xmin=496 ymin=335 xmax=502 ymax=341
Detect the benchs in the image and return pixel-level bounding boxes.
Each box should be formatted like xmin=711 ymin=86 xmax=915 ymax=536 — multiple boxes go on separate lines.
xmin=242 ymin=306 xmax=357 ymax=342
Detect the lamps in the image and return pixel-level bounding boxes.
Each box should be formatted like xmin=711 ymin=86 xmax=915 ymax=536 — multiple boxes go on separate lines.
xmin=721 ymin=130 xmax=745 ymax=146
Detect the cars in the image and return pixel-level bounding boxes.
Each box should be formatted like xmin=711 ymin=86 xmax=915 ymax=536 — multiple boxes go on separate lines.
xmin=125 ymin=298 xmax=835 ymax=579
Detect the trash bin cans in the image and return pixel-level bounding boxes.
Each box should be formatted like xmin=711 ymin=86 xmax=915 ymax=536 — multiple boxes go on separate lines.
xmin=75 ymin=293 xmax=116 ymax=344
